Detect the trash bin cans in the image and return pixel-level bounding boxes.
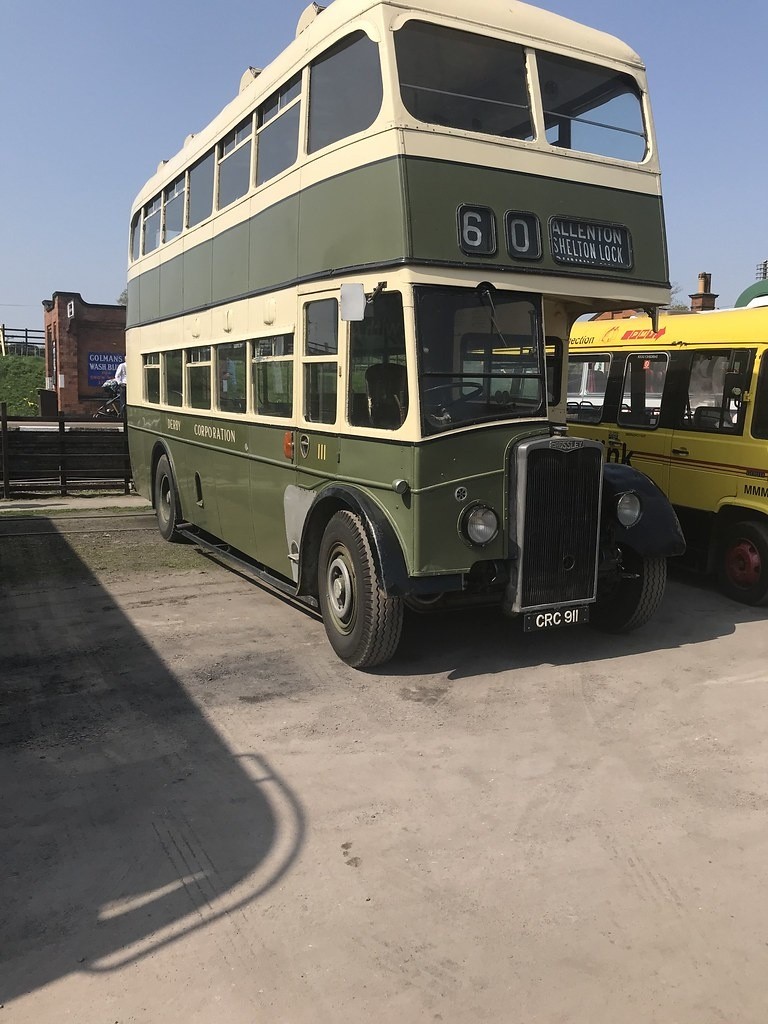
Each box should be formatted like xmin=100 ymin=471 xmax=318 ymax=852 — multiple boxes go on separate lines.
xmin=35 ymin=388 xmax=58 ymax=417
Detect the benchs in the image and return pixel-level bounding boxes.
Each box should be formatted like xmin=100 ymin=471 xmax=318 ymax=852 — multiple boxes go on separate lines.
xmin=561 ymin=401 xmax=734 ymax=429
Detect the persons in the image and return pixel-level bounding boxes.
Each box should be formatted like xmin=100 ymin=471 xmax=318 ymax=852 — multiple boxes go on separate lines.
xmin=114 ymin=355 xmax=126 ymax=413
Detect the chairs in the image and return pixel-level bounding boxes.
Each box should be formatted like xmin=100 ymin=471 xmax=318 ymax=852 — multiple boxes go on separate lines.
xmin=366 ymin=362 xmax=408 ymax=430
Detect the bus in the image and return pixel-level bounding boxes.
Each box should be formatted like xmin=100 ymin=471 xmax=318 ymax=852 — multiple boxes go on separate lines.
xmin=461 ymin=308 xmax=768 ymax=604
xmin=123 ymin=0 xmax=686 ymax=674
